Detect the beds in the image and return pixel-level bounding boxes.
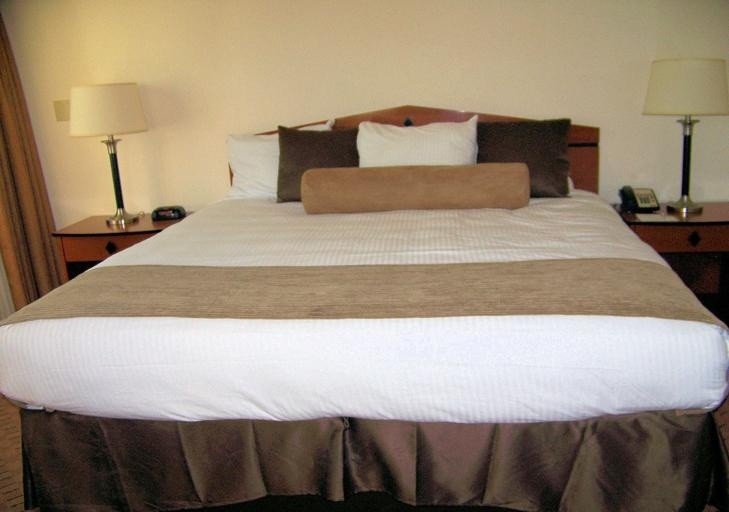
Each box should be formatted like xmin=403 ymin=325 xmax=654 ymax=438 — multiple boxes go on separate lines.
xmin=2 ymin=99 xmax=729 ymax=510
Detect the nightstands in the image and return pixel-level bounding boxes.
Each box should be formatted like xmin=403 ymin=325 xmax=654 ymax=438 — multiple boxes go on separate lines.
xmin=610 ymin=199 xmax=728 ymax=316
xmin=48 ymin=209 xmax=191 ymax=290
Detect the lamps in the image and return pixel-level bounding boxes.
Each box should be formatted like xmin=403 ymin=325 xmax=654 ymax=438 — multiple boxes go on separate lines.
xmin=642 ymin=56 xmax=729 ymax=216
xmin=67 ymin=79 xmax=148 ymax=226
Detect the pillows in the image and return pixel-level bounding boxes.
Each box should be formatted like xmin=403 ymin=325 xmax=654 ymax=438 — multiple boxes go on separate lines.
xmin=223 ymin=116 xmax=335 ymax=200
xmin=273 ymin=123 xmax=358 ymax=202
xmin=299 ymin=161 xmax=531 ymax=215
xmin=477 ymin=117 xmax=572 ymax=199
xmin=355 ymin=115 xmax=480 ymax=169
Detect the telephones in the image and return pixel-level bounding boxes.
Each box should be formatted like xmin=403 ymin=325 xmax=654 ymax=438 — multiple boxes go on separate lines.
xmin=620 ymin=185 xmax=660 ymax=213
